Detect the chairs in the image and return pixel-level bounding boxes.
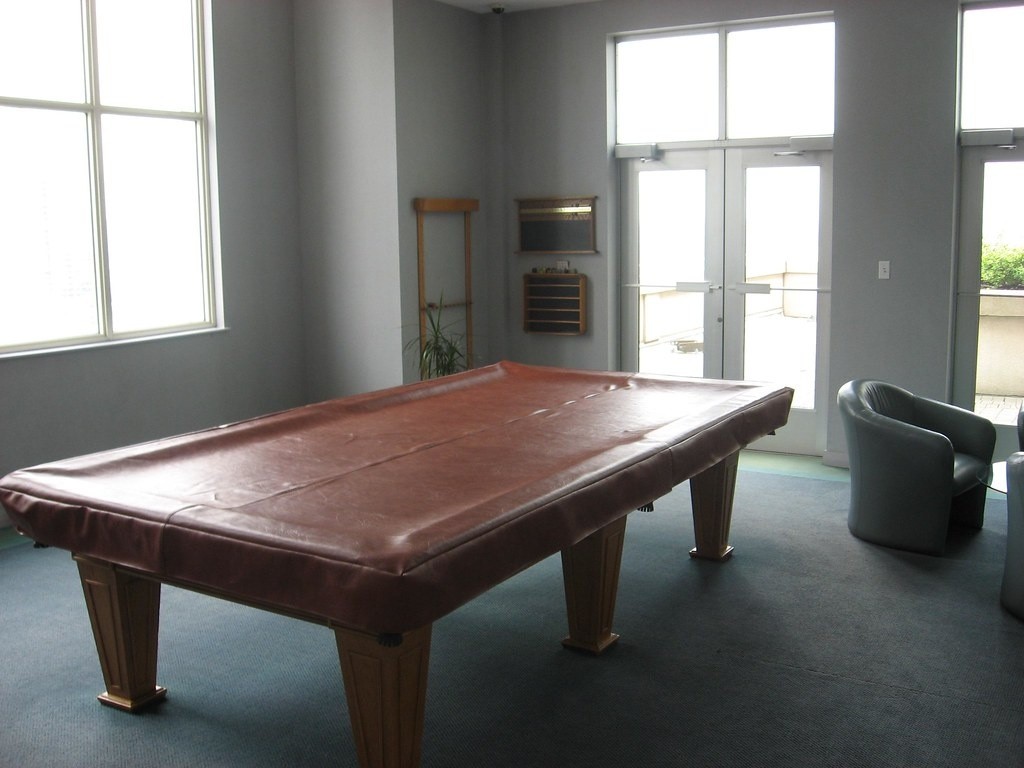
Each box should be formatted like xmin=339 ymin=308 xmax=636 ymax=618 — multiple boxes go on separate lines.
xmin=1000 ymin=451 xmax=1024 ymax=620
xmin=837 ymin=377 xmax=998 ymax=554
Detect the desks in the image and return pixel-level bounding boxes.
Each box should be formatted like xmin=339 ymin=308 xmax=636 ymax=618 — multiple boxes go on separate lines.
xmin=0 ymin=360 xmax=793 ymax=768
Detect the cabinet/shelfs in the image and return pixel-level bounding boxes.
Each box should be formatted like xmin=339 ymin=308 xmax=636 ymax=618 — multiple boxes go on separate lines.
xmin=523 ymin=272 xmax=586 ymax=336
